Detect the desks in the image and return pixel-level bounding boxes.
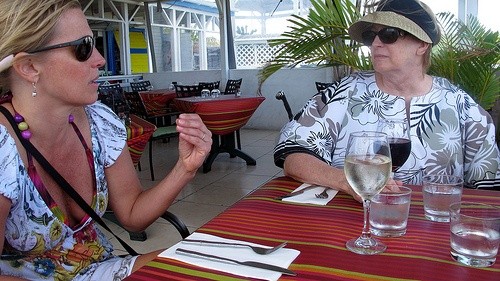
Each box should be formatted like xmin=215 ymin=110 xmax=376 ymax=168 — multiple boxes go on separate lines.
xmin=172 ymin=92 xmax=266 ymax=172
xmin=115 ymin=112 xmax=157 ymax=171
xmin=137 ymin=87 xmax=200 ymax=178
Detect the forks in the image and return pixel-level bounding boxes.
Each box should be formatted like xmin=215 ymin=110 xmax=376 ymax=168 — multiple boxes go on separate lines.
xmin=314 ymin=187 xmax=332 ymax=199
xmin=181 ymin=238 xmax=289 ymax=255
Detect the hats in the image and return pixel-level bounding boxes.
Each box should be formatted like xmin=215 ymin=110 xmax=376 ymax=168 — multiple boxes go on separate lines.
xmin=348 ymin=1 xmax=441 ymax=47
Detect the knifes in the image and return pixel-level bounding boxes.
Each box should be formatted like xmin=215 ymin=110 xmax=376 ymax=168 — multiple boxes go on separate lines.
xmin=176 ymin=248 xmax=297 ymax=277
xmin=275 ymin=183 xmax=319 ymax=199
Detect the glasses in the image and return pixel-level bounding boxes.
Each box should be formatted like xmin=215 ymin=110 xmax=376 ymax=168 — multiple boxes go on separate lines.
xmin=13 ymin=35 xmax=97 ymax=62
xmin=362 ymin=28 xmax=409 ymax=46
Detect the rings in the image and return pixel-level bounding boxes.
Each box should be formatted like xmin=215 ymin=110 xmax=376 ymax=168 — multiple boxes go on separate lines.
xmin=201 ymin=134 xmax=206 ymax=140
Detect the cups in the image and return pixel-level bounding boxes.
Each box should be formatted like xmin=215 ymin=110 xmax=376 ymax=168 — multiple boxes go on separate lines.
xmin=169 ymin=84 xmax=175 ymax=91
xmin=422 ymin=175 xmax=464 ymax=223
xmin=448 ymin=202 xmax=500 ymax=268
xmin=211 ymin=89 xmax=220 ymax=99
xmin=201 ymin=89 xmax=210 ymax=99
xmin=368 ymin=185 xmax=411 ymax=238
xmin=148 ymin=86 xmax=153 ymax=91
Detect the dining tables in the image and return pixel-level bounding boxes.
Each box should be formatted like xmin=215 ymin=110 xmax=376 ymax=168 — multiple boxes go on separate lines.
xmin=111 ymin=171 xmax=500 ymax=281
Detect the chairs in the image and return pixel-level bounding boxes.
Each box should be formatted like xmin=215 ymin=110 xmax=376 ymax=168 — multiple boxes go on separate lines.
xmin=174 ymin=84 xmax=201 ymax=99
xmin=314 ymin=79 xmax=337 ymax=92
xmin=198 ymin=80 xmax=221 ymax=92
xmin=99 ymin=83 xmax=131 ymax=119
xmin=221 ymin=77 xmax=244 ymax=150
xmin=131 ymin=80 xmax=164 ymax=127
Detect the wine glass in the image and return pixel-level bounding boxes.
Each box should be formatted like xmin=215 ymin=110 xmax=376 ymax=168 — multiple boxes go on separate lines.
xmin=373 ymin=119 xmax=412 ymax=197
xmin=344 ymin=131 xmax=392 ymax=256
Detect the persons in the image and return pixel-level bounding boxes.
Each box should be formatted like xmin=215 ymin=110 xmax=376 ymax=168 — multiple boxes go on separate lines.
xmin=272 ymin=1 xmax=500 ymax=205
xmin=0 ymin=0 xmax=214 ymax=281
